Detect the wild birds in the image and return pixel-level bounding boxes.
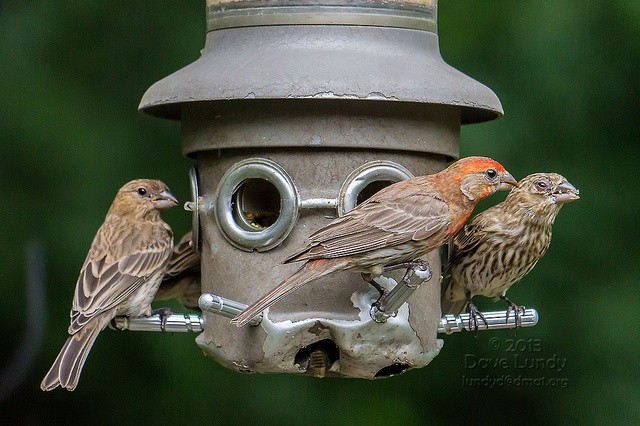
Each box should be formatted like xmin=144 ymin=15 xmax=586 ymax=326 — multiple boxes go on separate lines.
xmin=436 ymin=173 xmax=581 ymax=334
xmin=152 ymin=231 xmax=201 ymax=313
xmin=229 ymin=156 xmax=521 ymax=328
xmin=38 ymin=178 xmax=179 ymax=390
